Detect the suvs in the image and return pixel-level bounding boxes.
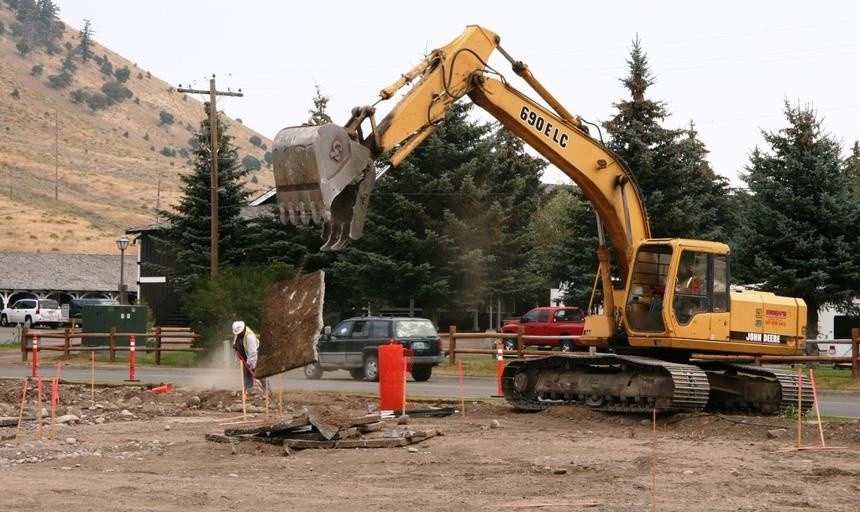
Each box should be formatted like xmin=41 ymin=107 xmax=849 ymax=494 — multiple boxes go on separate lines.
xmin=305 ymin=312 xmax=445 ymax=382
xmin=0 ymin=299 xmax=63 ymax=328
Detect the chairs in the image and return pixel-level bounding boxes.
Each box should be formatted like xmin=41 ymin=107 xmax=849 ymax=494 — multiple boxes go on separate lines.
xmin=540 ymin=313 xmax=571 ymax=321
xmin=351 ymin=330 xmax=389 ymax=337
xmin=683 ymin=277 xmax=703 ymax=294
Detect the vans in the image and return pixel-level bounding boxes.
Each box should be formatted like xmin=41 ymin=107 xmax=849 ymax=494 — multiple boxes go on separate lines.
xmin=69 ymin=298 xmax=121 ymax=327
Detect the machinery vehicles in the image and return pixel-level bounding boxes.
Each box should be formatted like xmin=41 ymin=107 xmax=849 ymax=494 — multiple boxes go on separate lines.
xmin=267 ymin=25 xmax=816 ymax=422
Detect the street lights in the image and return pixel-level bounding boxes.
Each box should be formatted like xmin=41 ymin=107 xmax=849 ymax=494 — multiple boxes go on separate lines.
xmin=115 ymin=237 xmax=129 ymax=284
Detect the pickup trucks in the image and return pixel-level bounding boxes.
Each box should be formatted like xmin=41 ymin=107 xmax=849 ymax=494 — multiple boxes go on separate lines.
xmin=501 ymin=306 xmax=587 ymax=353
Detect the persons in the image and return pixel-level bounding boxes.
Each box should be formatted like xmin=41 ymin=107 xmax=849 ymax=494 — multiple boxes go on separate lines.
xmin=650 ymin=258 xmax=699 ymax=317
xmin=231 ymin=320 xmax=270 ymax=392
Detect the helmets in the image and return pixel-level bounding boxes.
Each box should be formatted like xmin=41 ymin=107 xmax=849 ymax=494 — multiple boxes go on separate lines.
xmin=232 ymin=321 xmax=245 ymax=335
